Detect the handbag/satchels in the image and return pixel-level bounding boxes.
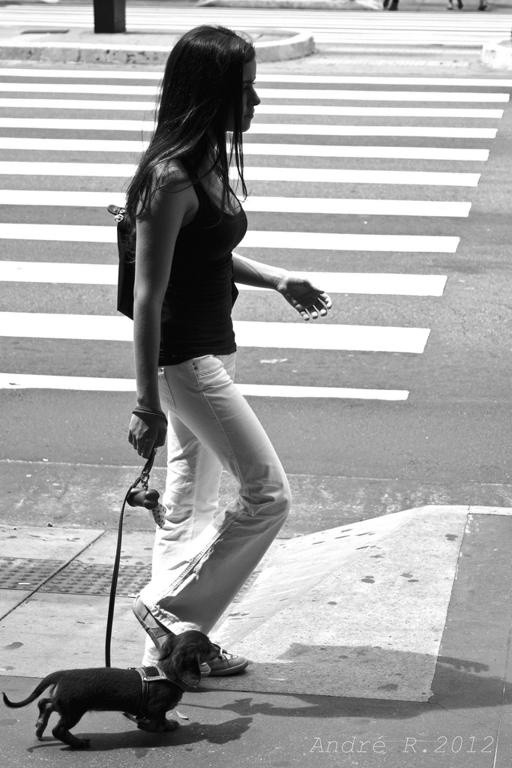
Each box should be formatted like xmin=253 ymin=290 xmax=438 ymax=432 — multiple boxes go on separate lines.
xmin=108 ymin=205 xmax=136 ymax=320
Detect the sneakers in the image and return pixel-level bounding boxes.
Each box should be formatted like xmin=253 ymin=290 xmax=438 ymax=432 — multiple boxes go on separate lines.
xmin=131 ymin=595 xmax=212 ymax=679
xmin=141 ymin=637 xmax=248 ymax=678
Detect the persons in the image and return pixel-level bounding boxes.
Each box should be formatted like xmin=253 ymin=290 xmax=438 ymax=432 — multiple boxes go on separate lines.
xmin=446 ymin=0 xmax=463 ymax=11
xmin=382 ymin=0 xmax=399 ymax=11
xmin=125 ymin=25 xmax=332 ymax=676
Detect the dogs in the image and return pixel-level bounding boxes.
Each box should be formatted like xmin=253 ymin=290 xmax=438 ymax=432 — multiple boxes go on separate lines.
xmin=2 ymin=629 xmax=221 ymax=747
